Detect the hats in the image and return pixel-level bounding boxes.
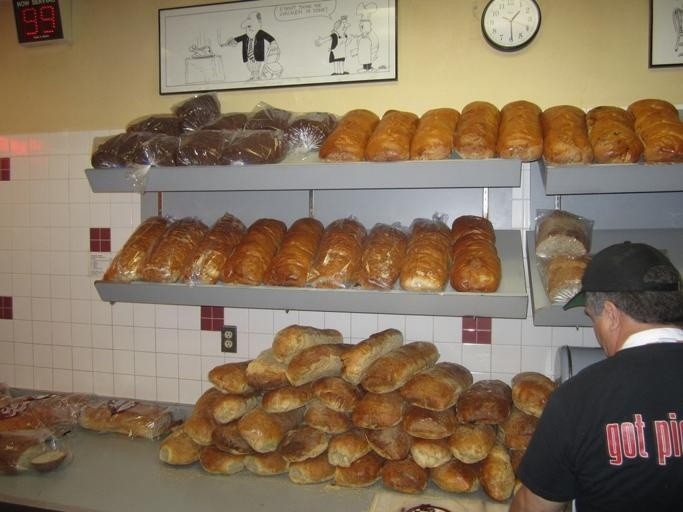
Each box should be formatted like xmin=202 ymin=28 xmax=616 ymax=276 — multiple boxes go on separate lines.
xmin=562 ymin=242 xmax=681 ymax=311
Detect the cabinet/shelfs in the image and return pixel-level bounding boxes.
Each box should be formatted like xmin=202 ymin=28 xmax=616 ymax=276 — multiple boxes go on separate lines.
xmin=85 ymin=108 xmax=683 ymax=328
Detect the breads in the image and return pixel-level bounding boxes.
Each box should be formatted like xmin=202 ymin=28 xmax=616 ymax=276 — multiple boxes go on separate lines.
xmin=160 ymin=326 xmax=561 ymax=501
xmin=410 ymin=108 xmax=459 ymax=160
xmin=585 ymin=105 xmax=644 ymax=165
xmin=454 ymin=100 xmax=500 ymax=159
xmin=365 ymin=110 xmax=419 ymax=162
xmin=88 ymin=94 xmax=336 ymax=166
xmin=400 ymin=212 xmax=451 ymax=293
xmin=533 ymin=211 xmax=593 ymax=304
xmin=354 ymin=221 xmax=408 ymax=291
xmin=263 ymin=217 xmax=325 ymax=287
xmin=220 ymin=217 xmax=288 ymax=286
xmin=451 ymin=215 xmax=502 ymax=292
xmin=138 ymin=215 xmax=209 ymax=283
xmin=307 ymin=215 xmax=365 ymax=289
xmin=627 ymin=99 xmax=683 ymax=164
xmin=319 ymin=109 xmax=378 ymax=162
xmin=541 ymin=105 xmax=594 ymax=166
xmin=0 ymin=381 xmax=183 ymax=477
xmin=104 ymin=214 xmax=175 ymax=283
xmin=180 ymin=212 xmax=248 ymax=285
xmin=499 ymin=100 xmax=542 ymax=163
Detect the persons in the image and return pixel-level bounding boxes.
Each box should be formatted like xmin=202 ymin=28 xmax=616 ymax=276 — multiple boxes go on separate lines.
xmin=500 ymin=240 xmax=682 ymax=510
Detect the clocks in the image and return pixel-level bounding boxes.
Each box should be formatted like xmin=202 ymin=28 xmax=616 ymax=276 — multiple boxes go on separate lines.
xmin=480 ymin=0 xmax=541 ymax=51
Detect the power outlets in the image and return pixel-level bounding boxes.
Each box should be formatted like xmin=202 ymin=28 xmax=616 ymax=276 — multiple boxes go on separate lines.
xmin=221 ymin=325 xmax=237 ymax=353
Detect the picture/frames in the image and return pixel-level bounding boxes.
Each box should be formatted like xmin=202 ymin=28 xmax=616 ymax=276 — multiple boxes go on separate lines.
xmin=157 ymin=0 xmax=397 ymax=95
xmin=649 ymin=0 xmax=683 ymax=69
